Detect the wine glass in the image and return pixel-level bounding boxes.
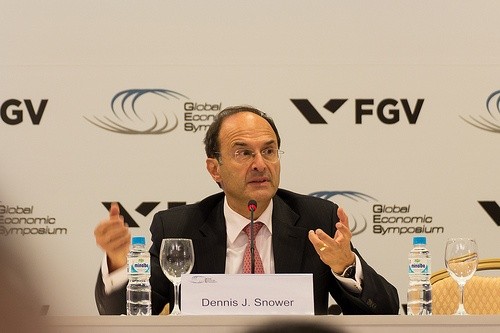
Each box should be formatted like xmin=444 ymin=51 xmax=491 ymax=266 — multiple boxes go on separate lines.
xmin=159 ymin=238 xmax=194 ymax=316
xmin=444 ymin=238 xmax=479 ymax=316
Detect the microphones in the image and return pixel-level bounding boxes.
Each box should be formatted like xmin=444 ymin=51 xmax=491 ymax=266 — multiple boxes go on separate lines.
xmin=248 ymin=199 xmax=257 ymax=273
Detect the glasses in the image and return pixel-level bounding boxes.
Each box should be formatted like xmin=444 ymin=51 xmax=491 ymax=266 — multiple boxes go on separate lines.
xmin=214 ymin=148 xmax=284 ymax=163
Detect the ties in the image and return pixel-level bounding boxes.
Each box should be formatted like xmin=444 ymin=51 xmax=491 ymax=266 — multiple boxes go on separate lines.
xmin=242 ymin=222 xmax=264 ymax=273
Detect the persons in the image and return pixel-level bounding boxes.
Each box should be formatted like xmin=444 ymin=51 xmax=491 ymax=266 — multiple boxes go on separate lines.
xmin=94 ymin=106 xmax=399 ymax=315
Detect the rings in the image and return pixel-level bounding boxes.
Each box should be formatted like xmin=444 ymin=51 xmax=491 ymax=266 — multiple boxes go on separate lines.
xmin=320 ymin=244 xmax=326 ymax=251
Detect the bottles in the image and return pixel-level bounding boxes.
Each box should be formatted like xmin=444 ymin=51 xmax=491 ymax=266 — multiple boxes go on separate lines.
xmin=406 ymin=236 xmax=433 ymax=317
xmin=125 ymin=236 xmax=152 ymax=317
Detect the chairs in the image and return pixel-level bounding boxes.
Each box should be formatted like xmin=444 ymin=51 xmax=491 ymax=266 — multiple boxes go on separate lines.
xmin=407 ymin=258 xmax=500 ymax=315
